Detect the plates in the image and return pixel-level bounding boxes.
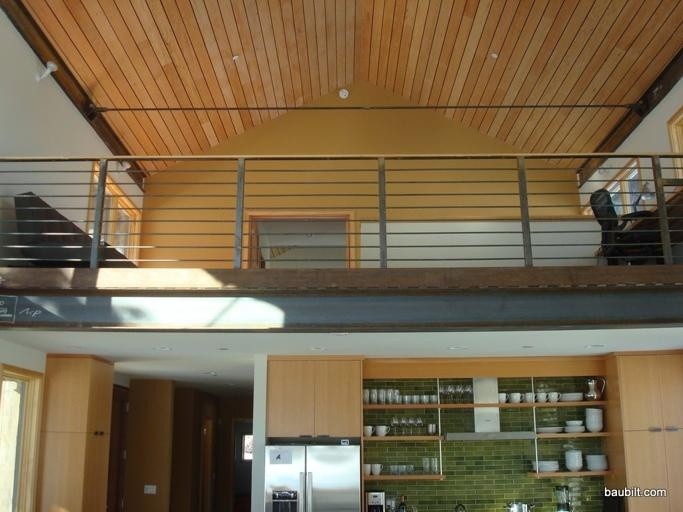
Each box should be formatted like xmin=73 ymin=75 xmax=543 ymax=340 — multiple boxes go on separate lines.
xmin=437 ymin=376 xmax=535 ymax=442
xmin=587 ymin=454 xmax=609 ymax=471
xmin=530 ymin=460 xmax=560 ymax=472
xmin=536 ymin=426 xmax=564 ymax=433
xmin=559 ymin=392 xmax=583 ymax=402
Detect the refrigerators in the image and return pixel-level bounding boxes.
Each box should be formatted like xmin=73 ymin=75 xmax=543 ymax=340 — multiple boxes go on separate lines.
xmin=263 ymin=435 xmax=360 ymax=512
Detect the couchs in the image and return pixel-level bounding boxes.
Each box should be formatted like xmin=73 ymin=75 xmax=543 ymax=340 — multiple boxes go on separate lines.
xmin=13 ymin=190 xmax=138 ymax=269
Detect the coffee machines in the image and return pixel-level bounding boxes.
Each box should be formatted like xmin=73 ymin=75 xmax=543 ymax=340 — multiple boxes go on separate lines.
xmin=553 ymin=484 xmax=574 ymax=511
xmin=363 ymin=490 xmax=385 ymax=512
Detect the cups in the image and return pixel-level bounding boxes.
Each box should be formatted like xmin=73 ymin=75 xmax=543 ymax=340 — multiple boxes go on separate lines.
xmin=361 ymin=462 xmax=383 ymax=474
xmin=497 ymin=391 xmax=561 ymax=404
xmin=360 ymin=389 xmax=437 ymax=402
xmin=422 ymin=456 xmax=439 ymax=475
xmin=426 ymin=423 xmax=437 ymax=435
xmin=364 ymin=425 xmax=390 ymax=436
xmin=389 ymin=462 xmax=415 ymax=475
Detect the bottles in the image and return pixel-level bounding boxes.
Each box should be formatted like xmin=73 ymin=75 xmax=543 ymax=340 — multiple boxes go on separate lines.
xmin=399 ymin=494 xmax=409 ymax=511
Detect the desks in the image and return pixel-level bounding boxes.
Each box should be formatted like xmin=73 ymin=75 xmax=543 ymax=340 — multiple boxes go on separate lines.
xmin=588 ymin=185 xmax=683 ymax=263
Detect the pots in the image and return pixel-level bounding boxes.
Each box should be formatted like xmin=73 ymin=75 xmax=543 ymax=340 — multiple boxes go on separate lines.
xmin=504 ymin=499 xmax=536 ymax=511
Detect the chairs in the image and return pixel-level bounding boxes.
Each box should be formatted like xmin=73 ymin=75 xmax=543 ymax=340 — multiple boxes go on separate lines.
xmin=589 ymin=188 xmax=661 ymax=265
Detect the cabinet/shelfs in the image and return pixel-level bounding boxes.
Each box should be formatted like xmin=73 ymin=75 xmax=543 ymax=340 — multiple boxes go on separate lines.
xmin=267 ymin=361 xmax=363 ymax=437
xmin=362 ymin=362 xmax=611 ymax=483
xmin=612 ymin=356 xmax=683 ymax=512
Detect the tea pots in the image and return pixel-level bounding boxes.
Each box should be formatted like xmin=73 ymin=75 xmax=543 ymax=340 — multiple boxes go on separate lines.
xmin=454 ymin=503 xmax=466 ymax=511
xmin=583 ymin=376 xmax=606 ymax=400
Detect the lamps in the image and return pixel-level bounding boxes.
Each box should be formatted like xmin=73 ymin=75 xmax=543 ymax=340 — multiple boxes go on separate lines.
xmin=35 ymin=60 xmax=57 ymax=82
xmin=116 ymin=159 xmax=132 ymax=175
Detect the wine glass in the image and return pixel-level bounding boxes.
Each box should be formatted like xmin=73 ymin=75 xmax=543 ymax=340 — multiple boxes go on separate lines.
xmin=390 ymin=415 xmax=423 ymax=437
xmin=438 ymin=384 xmax=472 ymax=403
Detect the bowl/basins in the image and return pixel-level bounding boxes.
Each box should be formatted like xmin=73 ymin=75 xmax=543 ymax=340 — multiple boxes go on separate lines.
xmin=565 ymin=450 xmax=583 ymax=473
xmin=563 ymin=425 xmax=585 ymax=432
xmin=565 ymin=419 xmax=584 ymax=426
xmin=584 ymin=407 xmax=603 ymax=432
